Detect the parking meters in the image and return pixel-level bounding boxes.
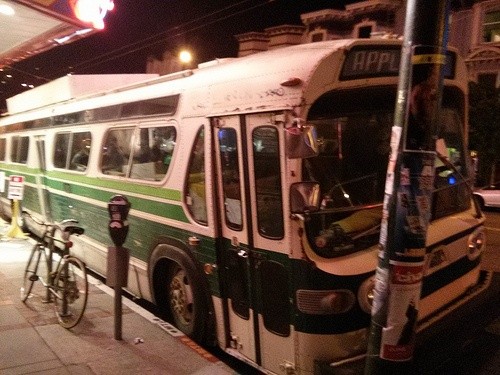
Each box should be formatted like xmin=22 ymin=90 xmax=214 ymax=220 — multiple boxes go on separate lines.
xmin=106 ymin=194 xmax=131 ymax=340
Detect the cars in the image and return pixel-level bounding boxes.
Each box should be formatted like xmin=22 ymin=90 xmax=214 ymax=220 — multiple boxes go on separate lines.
xmin=473 ymin=184 xmax=500 ymax=208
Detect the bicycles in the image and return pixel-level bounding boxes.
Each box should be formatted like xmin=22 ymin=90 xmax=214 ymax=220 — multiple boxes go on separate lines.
xmin=20 ymin=210 xmax=90 ymax=329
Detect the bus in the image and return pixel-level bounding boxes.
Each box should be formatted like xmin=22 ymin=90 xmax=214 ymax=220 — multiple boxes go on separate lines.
xmin=1 ymin=39 xmax=493 ymax=374
